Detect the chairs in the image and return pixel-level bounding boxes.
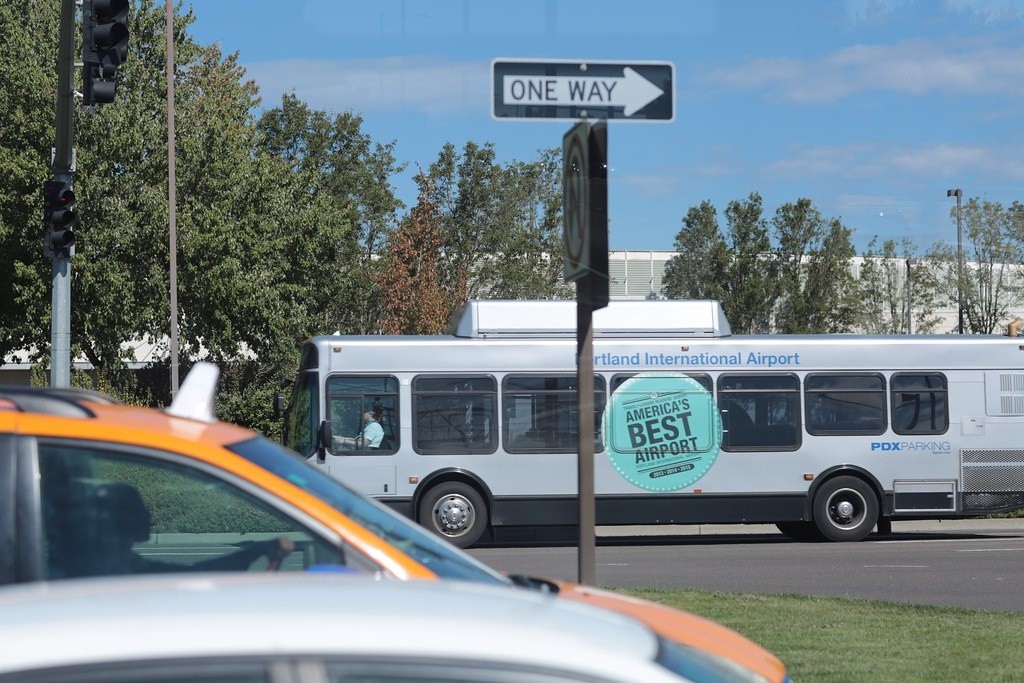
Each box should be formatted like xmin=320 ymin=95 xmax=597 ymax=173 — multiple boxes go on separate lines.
xmin=381 ymin=414 xmax=397 ymax=450
xmin=761 ymin=393 xmax=939 ymax=447
xmin=41 ymin=448 xmax=107 ymax=575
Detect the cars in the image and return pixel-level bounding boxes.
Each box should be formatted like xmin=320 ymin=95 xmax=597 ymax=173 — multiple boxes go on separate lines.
xmin=1 ymin=389 xmax=785 ymax=683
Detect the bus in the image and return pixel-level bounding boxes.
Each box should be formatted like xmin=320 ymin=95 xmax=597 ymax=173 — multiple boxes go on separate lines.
xmin=273 ymin=300 xmax=1024 ymax=550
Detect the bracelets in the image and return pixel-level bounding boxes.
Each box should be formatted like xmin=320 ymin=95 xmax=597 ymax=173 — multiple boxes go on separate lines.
xmin=344 ymin=439 xmax=345 ymax=445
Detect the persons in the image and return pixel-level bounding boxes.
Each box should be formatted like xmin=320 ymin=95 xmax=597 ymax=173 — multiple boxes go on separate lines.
xmin=88 ymin=483 xmax=294 ymax=570
xmin=334 ymin=406 xmax=383 ymax=448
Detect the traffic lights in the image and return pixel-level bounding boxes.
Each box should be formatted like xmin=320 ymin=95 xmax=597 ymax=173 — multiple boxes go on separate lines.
xmin=82 ymin=0 xmax=130 ymax=105
xmin=43 ymin=180 xmax=76 ymax=258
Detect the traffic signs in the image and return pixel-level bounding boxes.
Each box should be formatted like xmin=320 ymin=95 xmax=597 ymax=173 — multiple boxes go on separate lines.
xmin=491 ymin=57 xmax=676 ymax=123
